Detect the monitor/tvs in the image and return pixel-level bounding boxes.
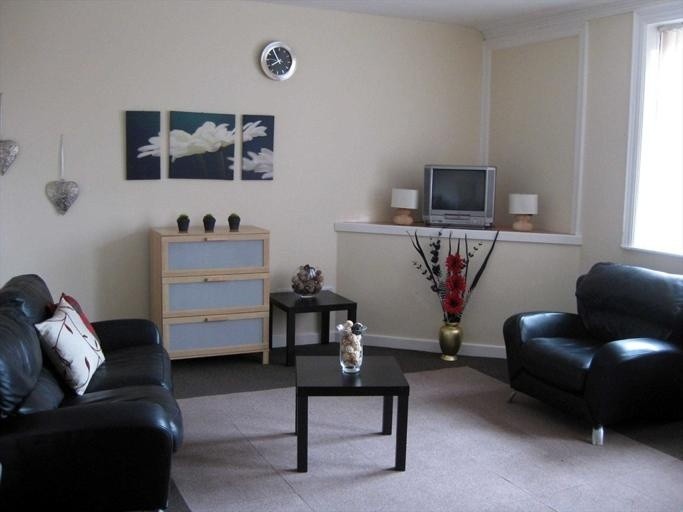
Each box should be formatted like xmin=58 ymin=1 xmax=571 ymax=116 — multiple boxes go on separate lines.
xmin=422 ymin=164 xmax=497 ymax=229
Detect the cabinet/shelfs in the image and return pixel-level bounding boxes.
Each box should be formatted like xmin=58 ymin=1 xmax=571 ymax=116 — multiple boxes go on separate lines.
xmin=147 ymin=223 xmax=272 ymax=366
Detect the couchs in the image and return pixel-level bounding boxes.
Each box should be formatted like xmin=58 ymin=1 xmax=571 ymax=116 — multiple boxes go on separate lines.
xmin=0 ymin=274 xmax=183 ymax=512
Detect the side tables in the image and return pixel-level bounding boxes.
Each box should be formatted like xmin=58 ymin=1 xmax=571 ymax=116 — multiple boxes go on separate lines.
xmin=296 ymin=355 xmax=409 ymax=473
xmin=268 ymin=291 xmax=357 ymax=367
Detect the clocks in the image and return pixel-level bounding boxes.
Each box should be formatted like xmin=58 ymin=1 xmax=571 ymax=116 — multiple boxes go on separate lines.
xmin=260 ymin=40 xmax=297 ymax=82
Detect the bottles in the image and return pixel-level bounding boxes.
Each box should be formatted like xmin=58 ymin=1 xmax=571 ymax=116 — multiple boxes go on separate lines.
xmin=336 ymin=321 xmax=368 ymax=375
xmin=341 ymin=371 xmax=361 ymax=387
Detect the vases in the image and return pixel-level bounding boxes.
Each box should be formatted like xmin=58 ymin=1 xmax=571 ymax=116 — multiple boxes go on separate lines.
xmin=291 ymin=263 xmax=325 ymax=298
xmin=339 ymin=325 xmax=368 ymax=374
xmin=438 ymin=318 xmax=463 ymax=362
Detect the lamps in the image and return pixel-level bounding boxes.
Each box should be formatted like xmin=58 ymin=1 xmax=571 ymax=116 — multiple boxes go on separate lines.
xmin=506 ymin=192 xmax=539 ymax=231
xmin=390 ymin=187 xmax=419 ymax=226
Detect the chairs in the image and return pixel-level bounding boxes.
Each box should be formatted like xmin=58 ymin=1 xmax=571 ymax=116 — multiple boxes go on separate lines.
xmin=503 ymin=261 xmax=683 ymax=446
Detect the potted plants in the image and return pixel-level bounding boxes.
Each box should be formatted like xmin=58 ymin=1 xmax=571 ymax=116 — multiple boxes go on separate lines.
xmin=177 ymin=214 xmax=190 ymax=232
xmin=228 ymin=213 xmax=241 ymax=232
xmin=202 ymin=213 xmax=216 ymax=232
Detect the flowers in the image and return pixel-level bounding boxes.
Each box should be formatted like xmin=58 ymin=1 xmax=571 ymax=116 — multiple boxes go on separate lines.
xmin=405 ymin=227 xmax=500 ymax=324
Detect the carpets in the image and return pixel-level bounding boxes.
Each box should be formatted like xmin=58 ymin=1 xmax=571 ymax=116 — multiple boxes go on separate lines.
xmin=169 ymin=365 xmax=683 ymax=511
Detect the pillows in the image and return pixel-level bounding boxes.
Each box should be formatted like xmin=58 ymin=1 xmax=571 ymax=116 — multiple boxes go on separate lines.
xmin=33 ymin=298 xmax=107 ymax=397
xmin=45 ymin=291 xmax=101 ymax=347
